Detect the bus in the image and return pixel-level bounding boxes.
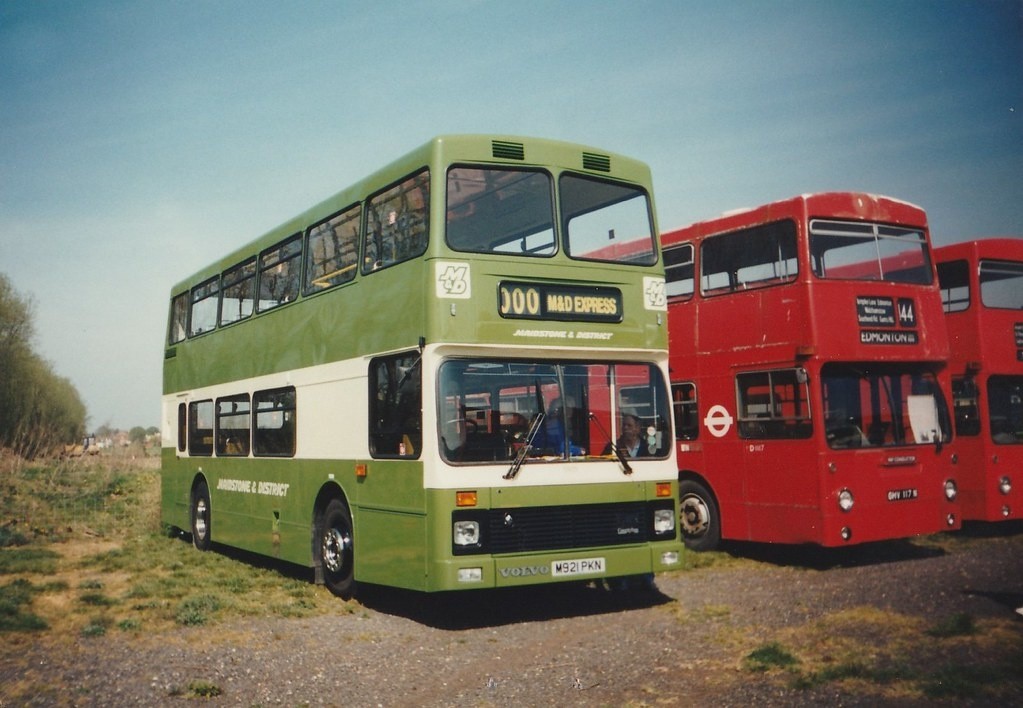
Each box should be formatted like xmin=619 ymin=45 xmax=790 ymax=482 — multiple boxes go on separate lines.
xmin=821 ymin=234 xmax=1022 ymax=535
xmin=155 ymin=129 xmax=691 ymax=597
xmin=444 ymin=191 xmax=964 ymax=550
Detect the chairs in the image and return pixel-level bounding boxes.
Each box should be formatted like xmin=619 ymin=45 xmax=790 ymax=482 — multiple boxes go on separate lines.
xmin=758 ymin=412 xmax=784 ymax=434
xmin=403 ymin=417 xmax=420 ymax=455
xmin=206 ymin=260 xmax=393 ymax=331
xmin=190 ymin=435 xmax=244 ymax=453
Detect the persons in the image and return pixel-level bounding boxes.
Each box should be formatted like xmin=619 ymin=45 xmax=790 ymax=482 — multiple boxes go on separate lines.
xmin=527 ymin=396 xmax=587 ymax=458
xmin=599 ymin=415 xmax=656 ymax=457
